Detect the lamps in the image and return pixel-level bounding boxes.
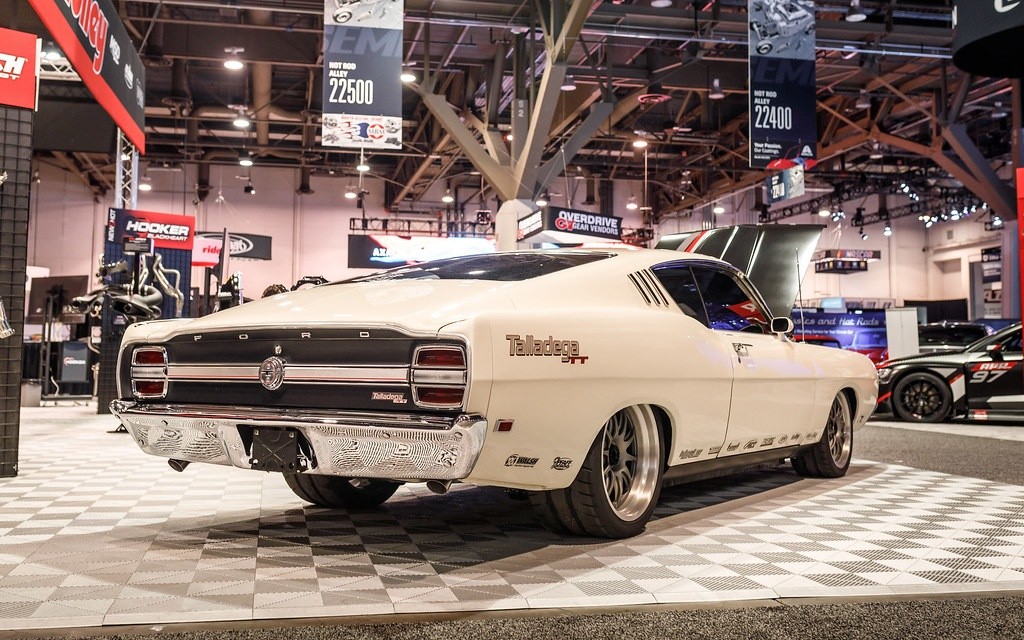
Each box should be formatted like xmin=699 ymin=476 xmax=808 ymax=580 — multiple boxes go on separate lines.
xmin=854 ymin=88 xmax=871 ymax=108
xmin=633 ymin=129 xmax=648 ymax=148
xmin=536 ymin=193 xmax=548 ymax=206
xmin=233 ymin=106 xmax=251 ymax=128
xmin=357 ymin=157 xmax=370 ymax=171
xmin=139 ymin=177 xmax=151 ymax=191
xmin=238 ymin=156 xmax=254 ymax=168
xmin=560 ymin=74 xmax=577 ymax=91
xmin=992 ymin=102 xmax=1008 ymax=118
xmin=344 ymin=185 xmax=357 ymax=198
xmin=441 ymin=188 xmax=453 ymax=203
xmin=709 ymin=78 xmax=724 ymax=100
xmin=401 ymin=61 xmax=417 ymax=82
xmin=844 ymin=0 xmax=867 ymax=22
xmin=224 ymin=46 xmax=245 ymax=69
xmin=830 ymin=179 xmax=1002 ymax=240
xmin=627 ymin=196 xmax=638 ymax=210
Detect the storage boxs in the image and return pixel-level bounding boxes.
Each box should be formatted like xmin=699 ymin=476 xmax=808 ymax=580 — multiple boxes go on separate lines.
xmin=58 ymin=340 xmax=90 ymax=384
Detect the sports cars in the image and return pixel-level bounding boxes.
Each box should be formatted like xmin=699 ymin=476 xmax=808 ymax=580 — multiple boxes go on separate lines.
xmin=877 ymin=324 xmax=1020 ymax=425
xmin=109 ymin=249 xmax=879 ymax=536
